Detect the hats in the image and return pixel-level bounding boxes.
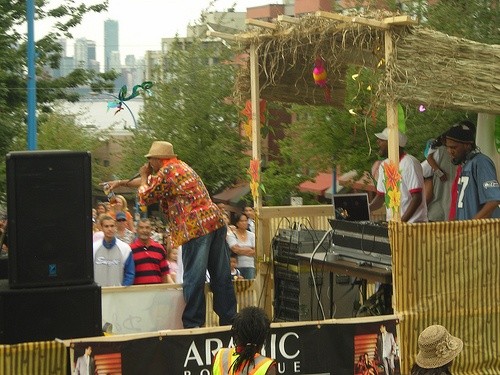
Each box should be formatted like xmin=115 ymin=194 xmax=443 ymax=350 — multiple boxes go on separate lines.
xmin=415 ymin=324 xmax=463 ymax=369
xmin=116 ymin=213 xmax=126 ymax=219
xmin=375 ymin=128 xmax=408 ymax=147
xmin=143 ymin=141 xmax=175 ymax=160
xmin=445 ymin=121 xmax=475 ymax=144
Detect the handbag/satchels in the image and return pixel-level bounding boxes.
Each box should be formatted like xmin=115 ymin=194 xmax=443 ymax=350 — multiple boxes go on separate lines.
xmin=225 ymin=230 xmax=237 ymax=257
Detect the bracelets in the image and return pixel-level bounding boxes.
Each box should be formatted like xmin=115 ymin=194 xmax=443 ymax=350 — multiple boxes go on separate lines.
xmin=118 ymin=179 xmax=121 ymax=185
xmin=434 ymin=168 xmax=445 ymax=178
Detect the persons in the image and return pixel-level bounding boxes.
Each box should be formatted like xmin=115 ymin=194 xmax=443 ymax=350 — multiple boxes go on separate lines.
xmin=212 ymin=305 xmax=278 ymax=375
xmin=407 ymin=324 xmax=464 ymax=375
xmin=73 ymin=344 xmax=97 ymax=375
xmin=356 ymin=352 xmax=375 ymax=375
xmin=420 ymin=120 xmax=500 ymax=223
xmin=90 ymin=195 xmax=211 ymax=287
xmin=375 ymin=324 xmax=397 ymax=375
xmin=0 ymin=219 xmax=9 ymax=280
xmin=369 ymin=128 xmax=428 ymax=306
xmin=96 ymin=138 xmax=237 ymax=329
xmin=215 ymin=202 xmax=256 ymax=279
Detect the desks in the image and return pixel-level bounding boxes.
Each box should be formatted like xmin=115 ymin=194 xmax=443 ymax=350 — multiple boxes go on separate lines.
xmin=296 ymin=253 xmax=392 ymax=318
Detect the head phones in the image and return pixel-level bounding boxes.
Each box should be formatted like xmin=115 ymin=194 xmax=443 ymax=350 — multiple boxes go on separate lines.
xmin=451 ymin=147 xmax=479 ymax=165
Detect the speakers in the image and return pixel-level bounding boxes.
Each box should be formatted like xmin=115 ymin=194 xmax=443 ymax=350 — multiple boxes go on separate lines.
xmin=0 ymin=280 xmax=104 ymax=346
xmin=6 ymin=150 xmax=94 ymax=288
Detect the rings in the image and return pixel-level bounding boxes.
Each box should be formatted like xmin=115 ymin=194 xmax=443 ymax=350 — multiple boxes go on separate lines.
xmin=103 ymin=181 xmax=110 ymax=190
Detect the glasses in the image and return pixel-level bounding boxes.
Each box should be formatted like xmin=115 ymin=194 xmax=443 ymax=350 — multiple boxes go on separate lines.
xmin=116 ymin=218 xmax=126 ymax=223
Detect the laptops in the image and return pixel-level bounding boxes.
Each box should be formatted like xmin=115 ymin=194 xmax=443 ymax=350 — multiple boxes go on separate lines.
xmin=333 ymin=193 xmax=370 ymax=225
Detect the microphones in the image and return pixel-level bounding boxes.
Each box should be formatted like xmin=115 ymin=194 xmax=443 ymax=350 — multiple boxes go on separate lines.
xmin=128 ymin=173 xmax=140 ymax=182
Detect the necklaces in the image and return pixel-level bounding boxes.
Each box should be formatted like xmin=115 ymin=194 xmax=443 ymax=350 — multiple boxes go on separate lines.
xmin=143 ymin=247 xmax=147 ymax=250
